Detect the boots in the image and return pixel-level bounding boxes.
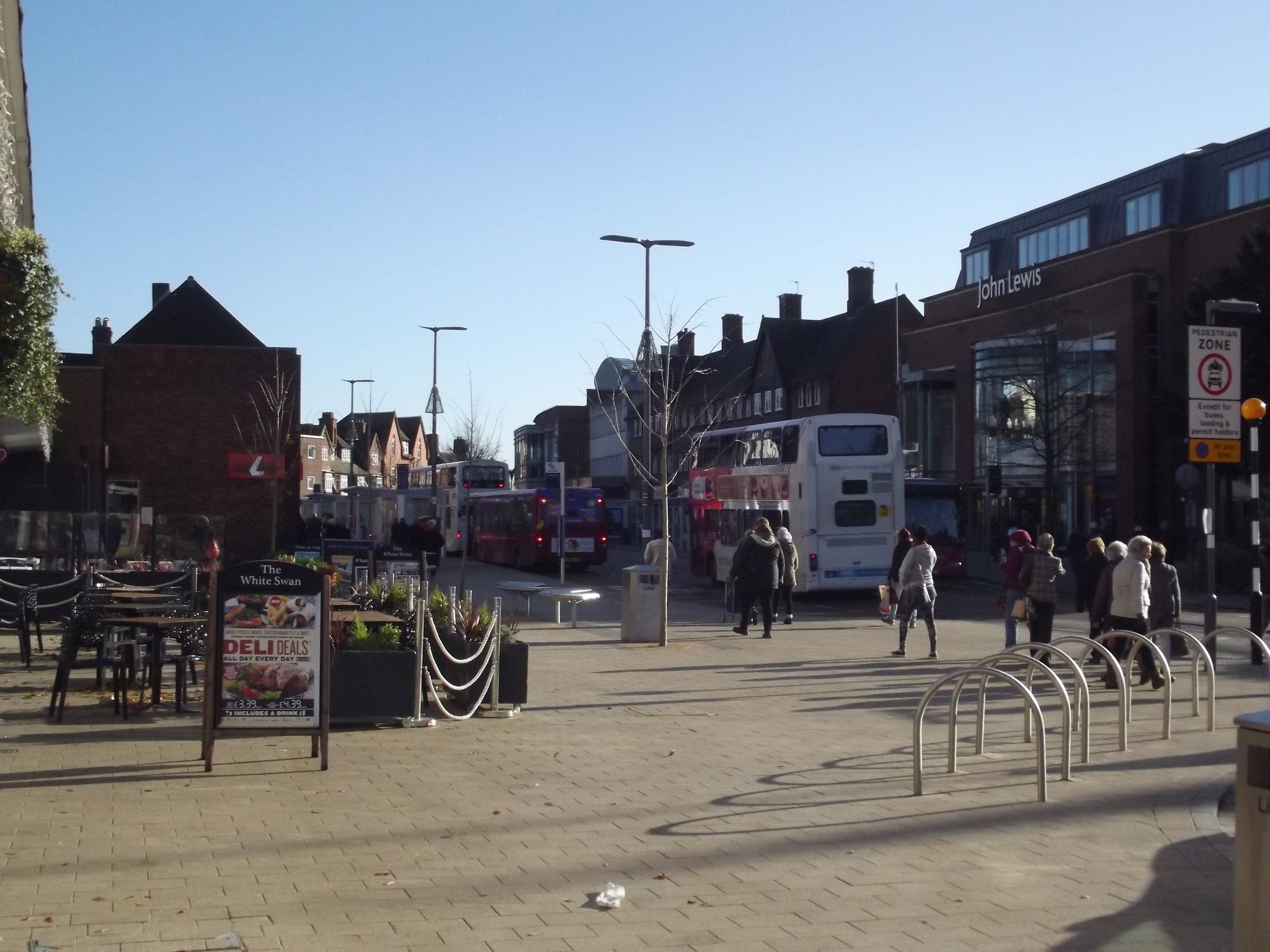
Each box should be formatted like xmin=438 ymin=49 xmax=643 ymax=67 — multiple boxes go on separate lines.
xmin=771 ymin=613 xmax=778 ymax=622
xmin=1105 ymin=657 xmax=1131 ymax=689
xmin=1146 ymin=659 xmax=1176 ymax=691
xmin=783 ymin=614 xmax=793 ymax=624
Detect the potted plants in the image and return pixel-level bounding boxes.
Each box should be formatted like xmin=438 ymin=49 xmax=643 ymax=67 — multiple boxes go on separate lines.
xmin=329 ymin=617 xmax=419 ymax=727
xmin=353 ymin=577 xmax=457 ymax=679
xmin=440 ymin=594 xmax=528 ymax=713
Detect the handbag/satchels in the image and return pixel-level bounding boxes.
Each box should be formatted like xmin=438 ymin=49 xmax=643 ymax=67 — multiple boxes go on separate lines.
xmin=878 ymin=585 xmax=891 ymax=615
xmin=1010 ymin=596 xmax=1028 ymax=623
xmin=904 ymin=584 xmax=931 ymax=609
xmin=1171 ymin=616 xmax=1190 ymax=658
xmin=886 ymin=580 xmax=899 ymax=605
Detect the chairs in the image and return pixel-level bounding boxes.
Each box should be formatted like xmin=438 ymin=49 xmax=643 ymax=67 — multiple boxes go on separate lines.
xmin=49 ymin=602 xmax=132 ymax=722
xmin=1 ymin=584 xmax=43 ymax=664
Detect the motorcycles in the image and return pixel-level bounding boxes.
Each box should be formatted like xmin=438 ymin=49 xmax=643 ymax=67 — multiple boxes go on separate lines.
xmin=1208 ymin=362 xmax=1223 ymax=372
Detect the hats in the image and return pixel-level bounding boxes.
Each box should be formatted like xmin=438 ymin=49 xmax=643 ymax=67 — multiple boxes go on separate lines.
xmin=1009 ymin=529 xmax=1032 ymax=544
xmin=898 ymin=527 xmax=913 ymax=542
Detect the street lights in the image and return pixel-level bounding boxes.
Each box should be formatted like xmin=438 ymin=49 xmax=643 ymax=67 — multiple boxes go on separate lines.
xmin=418 ymin=325 xmax=468 ymax=519
xmin=1055 ymin=305 xmax=1103 ymax=539
xmin=508 ymin=469 xmax=516 ymax=491
xmin=340 ymin=379 xmax=375 ymax=528
xmin=1203 ymin=294 xmax=1260 ymax=674
xmin=600 ymin=234 xmax=696 ymax=582
xmin=1241 ymin=397 xmax=1266 ymax=665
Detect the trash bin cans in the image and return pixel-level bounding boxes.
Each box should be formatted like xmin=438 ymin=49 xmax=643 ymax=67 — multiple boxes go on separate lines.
xmin=1233 ymin=711 xmax=1270 ymax=952
xmin=620 ymin=565 xmax=665 ymax=643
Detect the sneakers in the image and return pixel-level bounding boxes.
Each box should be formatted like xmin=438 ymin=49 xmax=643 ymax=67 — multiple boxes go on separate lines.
xmin=927 ymin=652 xmax=938 ymax=659
xmin=891 ymin=650 xmax=906 ymax=657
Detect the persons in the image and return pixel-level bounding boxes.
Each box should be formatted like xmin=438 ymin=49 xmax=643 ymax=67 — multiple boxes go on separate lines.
xmin=1000 ymin=530 xmax=1036 ymax=651
xmin=101 ymin=513 xmax=126 ymax=559
xmin=1105 ymin=534 xmax=1176 ymax=689
xmin=1083 ymin=537 xmax=1112 ymax=663
xmin=733 ymin=528 xmax=758 ymax=626
xmin=282 ymin=503 xmax=449 ymax=585
xmin=643 ymin=530 xmax=678 ymax=582
xmin=772 ymin=527 xmax=799 ymax=624
xmin=882 ymin=528 xmax=917 ymax=628
xmin=1090 ymin=541 xmax=1151 ymax=685
xmin=891 ymin=525 xmax=938 ymax=661
xmin=1019 ymin=534 xmax=1067 ymax=667
xmin=729 ymin=517 xmax=785 ymax=638
xmin=192 ymin=517 xmax=216 ymax=559
xmin=1147 ymin=541 xmax=1181 ymax=674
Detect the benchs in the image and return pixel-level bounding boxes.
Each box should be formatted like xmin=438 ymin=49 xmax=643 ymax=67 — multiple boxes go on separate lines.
xmin=494 ymin=581 xmax=600 ymax=629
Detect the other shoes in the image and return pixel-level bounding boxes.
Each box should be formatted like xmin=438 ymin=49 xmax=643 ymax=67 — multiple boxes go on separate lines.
xmin=732 ymin=626 xmax=749 ymax=636
xmin=761 ymin=631 xmax=773 ymax=638
xmin=1087 ymin=655 xmax=1103 ymax=664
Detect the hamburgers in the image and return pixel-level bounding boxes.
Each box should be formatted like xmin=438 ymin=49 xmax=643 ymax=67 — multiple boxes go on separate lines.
xmin=224 ymin=604 xmax=262 ymax=628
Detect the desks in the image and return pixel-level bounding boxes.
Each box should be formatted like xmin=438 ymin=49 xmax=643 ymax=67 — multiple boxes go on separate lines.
xmin=84 ymin=586 xmax=207 ymax=719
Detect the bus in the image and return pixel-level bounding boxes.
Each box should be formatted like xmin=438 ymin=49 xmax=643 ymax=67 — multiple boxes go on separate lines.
xmin=461 ymin=488 xmax=608 ymax=574
xmin=903 ymin=476 xmax=970 ymax=582
xmin=409 ymin=458 xmax=511 ymax=556
xmin=682 ymin=412 xmax=905 ymax=594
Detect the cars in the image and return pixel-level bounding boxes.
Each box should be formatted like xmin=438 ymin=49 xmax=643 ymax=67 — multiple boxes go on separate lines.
xmin=1207 ymin=371 xmax=1222 ymax=389
